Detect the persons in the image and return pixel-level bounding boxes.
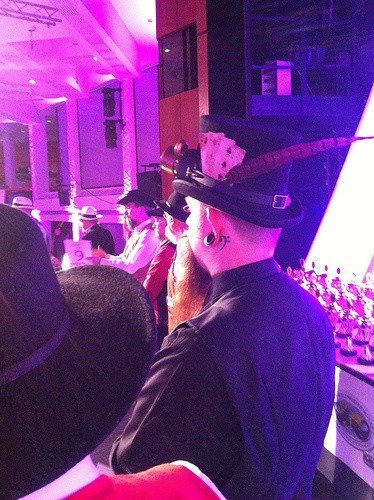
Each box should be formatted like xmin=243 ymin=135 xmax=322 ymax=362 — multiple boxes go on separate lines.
xmin=0 ymin=190 xmax=228 ymax=500
xmin=109 ymin=113 xmax=335 ymax=500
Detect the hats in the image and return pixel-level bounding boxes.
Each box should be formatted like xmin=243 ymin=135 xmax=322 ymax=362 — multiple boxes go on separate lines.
xmin=116 ymin=189 xmax=156 ymax=208
xmin=10 ymin=197 xmax=35 ymax=209
xmin=173 ymin=114 xmax=374 ymax=228
xmin=152 ymin=189 xmax=191 ymax=223
xmin=0 ymin=201 xmax=157 ymax=500
xmin=78 ymin=205 xmax=103 ymax=221
xmin=147 ymin=207 xmax=164 ymax=218
